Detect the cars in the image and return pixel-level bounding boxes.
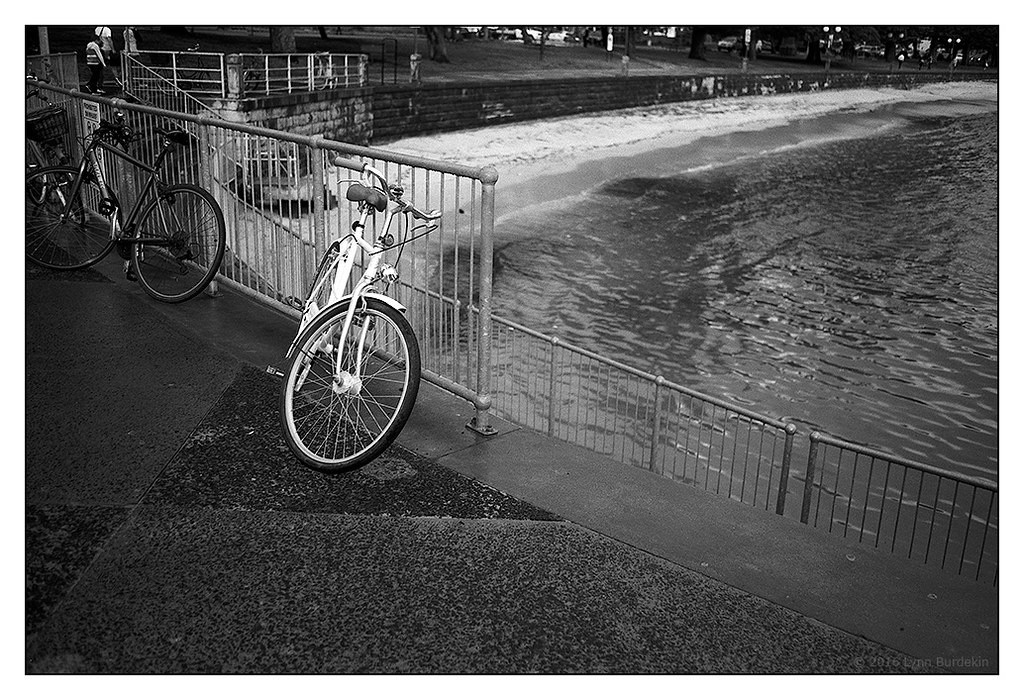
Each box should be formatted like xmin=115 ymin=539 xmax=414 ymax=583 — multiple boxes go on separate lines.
xmin=716 ymin=34 xmax=763 ymax=55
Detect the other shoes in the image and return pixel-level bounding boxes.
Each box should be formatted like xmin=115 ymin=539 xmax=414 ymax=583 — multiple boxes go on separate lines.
xmin=86 ymin=85 xmax=92 ymax=93
xmin=97 ymin=89 xmax=106 ymax=94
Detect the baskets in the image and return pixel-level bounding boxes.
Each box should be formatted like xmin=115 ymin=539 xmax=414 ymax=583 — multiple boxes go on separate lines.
xmin=26 ymin=107 xmax=70 ymax=142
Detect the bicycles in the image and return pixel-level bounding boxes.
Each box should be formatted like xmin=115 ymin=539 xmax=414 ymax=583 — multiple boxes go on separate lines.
xmin=152 ymin=41 xmax=228 ymax=99
xmin=264 ymin=155 xmax=445 ymax=477
xmin=309 ymin=52 xmax=338 ymax=91
xmin=242 ymin=47 xmax=265 ymax=96
xmin=25 ymin=84 xmax=228 ymax=305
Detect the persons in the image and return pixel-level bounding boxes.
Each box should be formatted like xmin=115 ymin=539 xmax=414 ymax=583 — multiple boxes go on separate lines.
xmin=121 ymin=29 xmax=140 ymax=61
xmin=243 ymin=47 xmax=265 ymax=90
xmin=917 ymin=47 xmax=933 ymax=70
xmin=408 ymin=51 xmax=423 ymax=84
xmin=83 ymin=26 xmax=116 ymax=95
xmin=895 ymin=47 xmax=907 ymax=69
xmin=425 ymin=26 xmax=441 ymax=59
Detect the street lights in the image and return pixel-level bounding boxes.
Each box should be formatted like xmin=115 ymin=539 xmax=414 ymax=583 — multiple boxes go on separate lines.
xmin=887 ymin=32 xmax=904 ymax=74
xmin=948 ymin=37 xmax=962 ymax=81
xmin=823 ymin=26 xmax=842 ymax=72
